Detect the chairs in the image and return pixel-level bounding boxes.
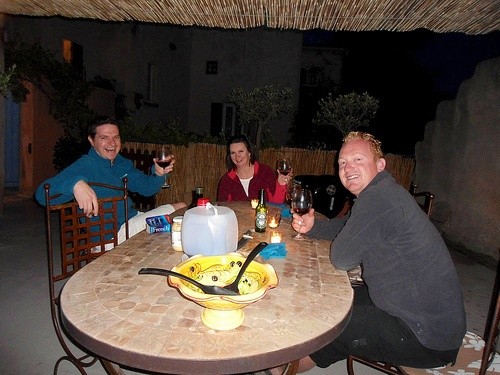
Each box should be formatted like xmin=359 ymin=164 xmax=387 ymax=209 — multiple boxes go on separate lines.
xmin=347 ymin=248 xmax=500 ymax=375
xmin=44 ymin=178 xmax=129 ymax=375
xmin=412 ymin=183 xmax=435 ymax=218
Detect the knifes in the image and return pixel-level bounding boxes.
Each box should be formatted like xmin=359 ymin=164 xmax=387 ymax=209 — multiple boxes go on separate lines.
xmin=237 ymin=229 xmax=253 ymax=250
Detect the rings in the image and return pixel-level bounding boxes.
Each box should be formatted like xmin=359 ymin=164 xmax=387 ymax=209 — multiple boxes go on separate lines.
xmin=285 ymin=178 xmax=287 ymax=182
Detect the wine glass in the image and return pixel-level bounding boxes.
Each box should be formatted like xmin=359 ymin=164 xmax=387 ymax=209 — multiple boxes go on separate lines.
xmin=156 ymin=141 xmax=175 ymax=189
xmin=277 ymin=158 xmax=294 ymax=194
xmin=291 ymin=189 xmax=313 ymax=241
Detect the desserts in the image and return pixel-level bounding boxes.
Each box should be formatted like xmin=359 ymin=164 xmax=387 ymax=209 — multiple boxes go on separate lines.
xmin=181 ymin=261 xmax=258 ymax=296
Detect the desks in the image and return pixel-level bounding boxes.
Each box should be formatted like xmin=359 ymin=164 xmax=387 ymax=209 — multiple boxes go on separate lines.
xmin=60 ymin=200 xmax=354 ymax=375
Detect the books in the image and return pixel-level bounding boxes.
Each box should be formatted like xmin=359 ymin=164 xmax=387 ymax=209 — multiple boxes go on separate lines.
xmin=145 ymin=215 xmax=173 ymax=234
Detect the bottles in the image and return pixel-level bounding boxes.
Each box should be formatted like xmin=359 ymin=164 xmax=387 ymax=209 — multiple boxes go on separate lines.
xmin=254 ymin=189 xmax=267 ymax=232
xmin=188 ymin=187 xmax=203 ymax=210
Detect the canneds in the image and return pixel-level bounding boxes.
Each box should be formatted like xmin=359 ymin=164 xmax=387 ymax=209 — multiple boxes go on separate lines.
xmin=171 ymin=216 xmax=183 ymax=252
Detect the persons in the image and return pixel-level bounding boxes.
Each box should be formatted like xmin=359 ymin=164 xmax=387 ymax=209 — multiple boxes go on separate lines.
xmin=35 ymin=114 xmax=188 ymax=262
xmin=216 ymin=134 xmax=293 ymax=204
xmin=267 ymin=130 xmax=467 ymax=375
